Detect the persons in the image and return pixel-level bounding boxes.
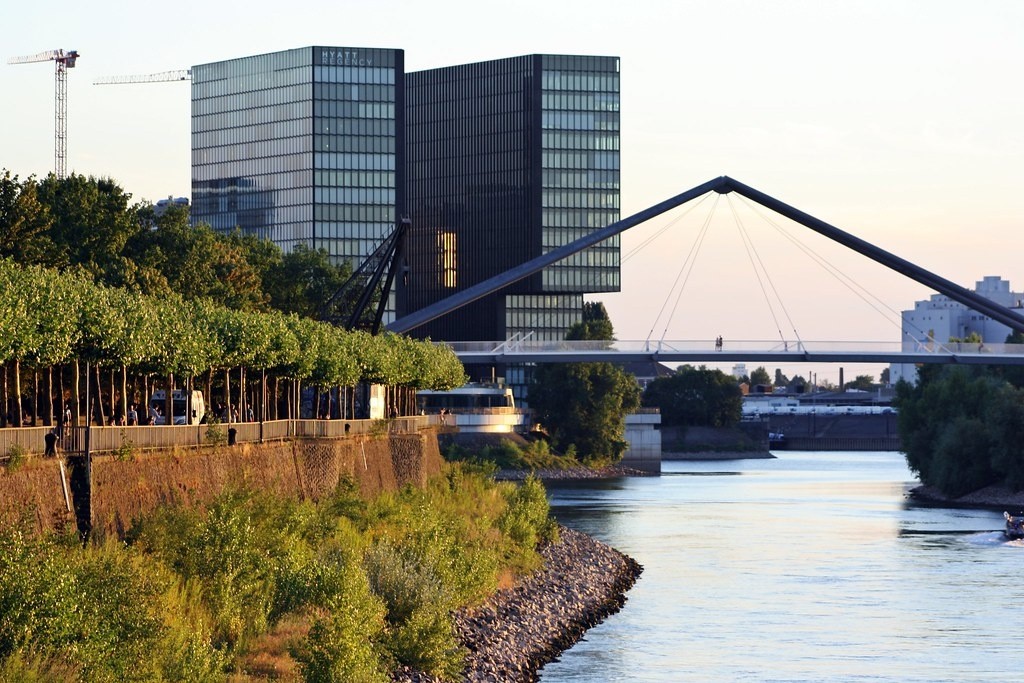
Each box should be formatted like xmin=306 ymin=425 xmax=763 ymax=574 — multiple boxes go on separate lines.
xmin=715 ymin=335 xmax=722 ymax=352
xmin=392 ymin=408 xmax=398 ymax=417
xmin=440 ymin=408 xmax=452 ymax=420
xmin=977 ymin=340 xmax=982 ymax=353
xmin=64 ymin=404 xmax=72 ymax=437
xmin=317 ymin=409 xmax=330 ymax=420
xmin=213 ymin=403 xmax=254 ymax=422
xmin=421 ymin=408 xmax=424 ymax=416
xmin=112 ymin=402 xmax=161 ymax=425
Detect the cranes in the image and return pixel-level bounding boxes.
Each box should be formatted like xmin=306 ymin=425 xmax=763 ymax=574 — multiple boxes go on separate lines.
xmin=5 ymin=45 xmax=80 ymax=180
xmin=92 ymin=66 xmax=191 ymax=85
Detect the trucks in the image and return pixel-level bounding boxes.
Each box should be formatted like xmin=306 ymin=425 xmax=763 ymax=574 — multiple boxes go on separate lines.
xmin=147 ymin=389 xmax=205 ymax=426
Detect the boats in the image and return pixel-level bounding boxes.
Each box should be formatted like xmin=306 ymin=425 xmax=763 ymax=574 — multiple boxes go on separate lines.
xmin=1005 ymin=523 xmax=1024 ymax=540
